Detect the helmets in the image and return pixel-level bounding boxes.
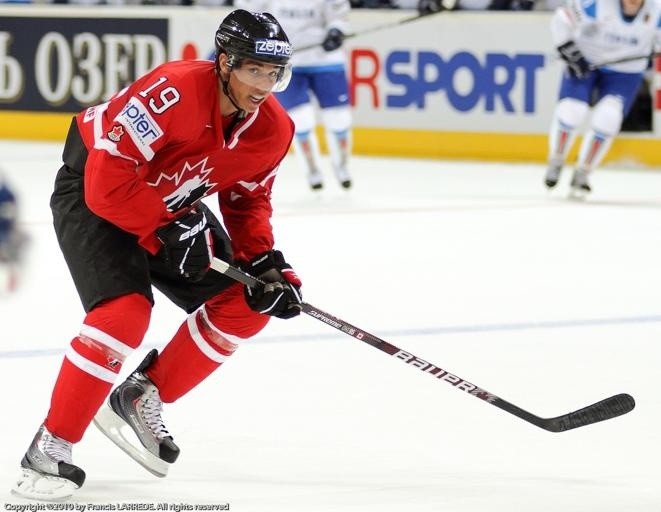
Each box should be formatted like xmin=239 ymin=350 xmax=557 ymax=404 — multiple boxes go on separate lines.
xmin=215 ymin=8 xmax=294 ymax=69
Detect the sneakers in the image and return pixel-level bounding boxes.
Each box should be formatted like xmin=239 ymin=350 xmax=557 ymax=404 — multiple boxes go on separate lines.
xmin=336 ymin=168 xmax=352 ymax=188
xmin=310 ymin=172 xmax=324 ymax=190
xmin=544 ymin=165 xmax=563 ymax=186
xmin=571 ymin=169 xmax=595 ymax=192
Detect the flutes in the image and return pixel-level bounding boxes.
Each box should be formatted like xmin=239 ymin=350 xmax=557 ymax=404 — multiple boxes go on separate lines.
xmin=209 ymin=256 xmax=635 ymax=432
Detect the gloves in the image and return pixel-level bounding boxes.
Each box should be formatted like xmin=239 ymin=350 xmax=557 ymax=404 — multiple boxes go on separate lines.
xmin=240 ymin=249 xmax=303 ymax=321
xmin=321 ymin=28 xmax=344 ymax=53
xmin=156 ymin=210 xmax=215 ymax=284
xmin=557 ymin=40 xmax=590 ymax=80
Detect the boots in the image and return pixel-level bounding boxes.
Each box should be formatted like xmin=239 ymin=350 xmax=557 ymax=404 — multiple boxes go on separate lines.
xmin=20 ymin=421 xmax=88 ymax=488
xmin=107 ymin=345 xmax=180 ymax=463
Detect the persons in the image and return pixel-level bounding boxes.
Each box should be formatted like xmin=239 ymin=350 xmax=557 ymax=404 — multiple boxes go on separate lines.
xmin=544 ymin=0 xmax=661 ymax=191
xmin=1 ymin=182 xmax=29 ymax=266
xmin=19 ymin=7 xmax=301 ymax=488
xmin=233 ymin=0 xmax=352 ymax=190
